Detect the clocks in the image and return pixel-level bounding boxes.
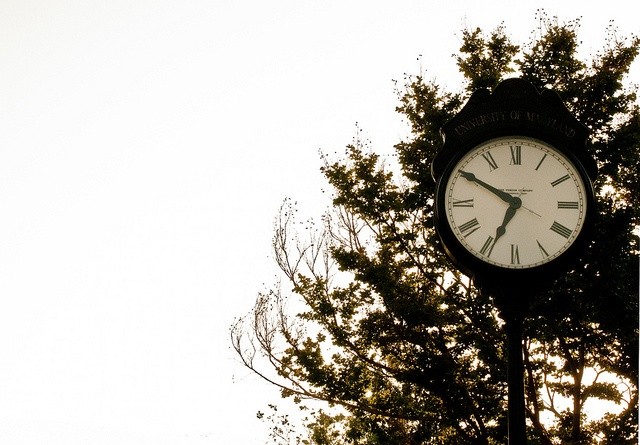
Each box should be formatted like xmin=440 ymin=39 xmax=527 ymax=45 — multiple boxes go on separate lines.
xmin=434 ymin=131 xmax=596 ymax=286
